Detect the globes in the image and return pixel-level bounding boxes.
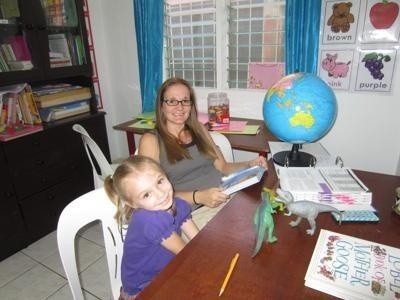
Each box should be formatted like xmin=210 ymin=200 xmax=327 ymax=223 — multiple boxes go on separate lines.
xmin=262 ymin=72 xmax=338 ymax=167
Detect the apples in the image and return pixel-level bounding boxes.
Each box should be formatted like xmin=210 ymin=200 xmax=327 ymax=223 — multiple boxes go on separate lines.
xmin=369 ymin=0 xmax=399 ymax=30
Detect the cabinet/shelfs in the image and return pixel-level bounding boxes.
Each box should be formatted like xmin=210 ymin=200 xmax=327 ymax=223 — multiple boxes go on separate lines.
xmin=0 ymin=0 xmax=113 ymax=263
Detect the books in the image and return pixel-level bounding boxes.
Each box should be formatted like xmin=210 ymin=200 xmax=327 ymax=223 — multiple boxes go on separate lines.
xmin=1 ymin=0 xmax=93 ymax=142
xmin=217 ymin=166 xmax=264 ymax=194
xmin=304 ymin=228 xmax=400 ymax=299
xmin=275 ymin=164 xmax=373 ymax=211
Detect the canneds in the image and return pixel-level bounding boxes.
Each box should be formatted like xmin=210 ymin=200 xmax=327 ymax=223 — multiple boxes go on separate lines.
xmin=207 ymin=92 xmax=230 ymax=128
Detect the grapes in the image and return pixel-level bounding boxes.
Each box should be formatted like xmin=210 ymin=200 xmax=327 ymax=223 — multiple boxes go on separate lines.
xmin=361 ymin=52 xmax=387 ymax=81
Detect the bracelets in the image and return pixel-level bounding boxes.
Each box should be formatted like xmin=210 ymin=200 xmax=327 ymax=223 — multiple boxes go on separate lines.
xmin=193 ymin=189 xmax=200 ymax=205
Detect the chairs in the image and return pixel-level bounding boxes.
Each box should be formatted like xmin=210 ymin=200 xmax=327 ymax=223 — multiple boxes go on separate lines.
xmin=56 ymin=188 xmax=131 ymax=300
xmin=207 ymin=131 xmax=234 ymax=164
xmin=72 ymin=123 xmax=131 ymax=191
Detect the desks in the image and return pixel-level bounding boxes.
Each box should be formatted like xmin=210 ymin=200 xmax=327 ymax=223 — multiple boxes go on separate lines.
xmin=113 ymin=116 xmax=286 ymax=162
xmin=128 ymin=165 xmax=400 ymax=300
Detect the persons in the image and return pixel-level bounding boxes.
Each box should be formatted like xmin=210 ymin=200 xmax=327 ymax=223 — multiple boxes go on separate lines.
xmin=138 ymin=77 xmax=268 ymax=243
xmin=103 ymin=156 xmax=201 ymax=300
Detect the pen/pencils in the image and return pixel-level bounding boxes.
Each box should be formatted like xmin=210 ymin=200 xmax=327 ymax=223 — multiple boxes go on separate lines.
xmin=218 ymin=252 xmax=240 ymax=297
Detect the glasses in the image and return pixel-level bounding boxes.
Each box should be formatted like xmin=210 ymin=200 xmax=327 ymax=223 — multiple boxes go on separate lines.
xmin=162 ymin=100 xmax=193 ymax=106
